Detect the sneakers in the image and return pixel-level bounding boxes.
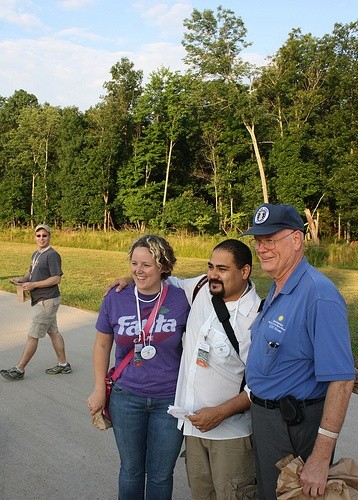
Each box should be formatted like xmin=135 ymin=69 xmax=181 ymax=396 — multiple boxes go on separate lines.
xmin=46 ymin=363 xmax=72 ymax=375
xmin=0 ymin=367 xmax=25 ymax=380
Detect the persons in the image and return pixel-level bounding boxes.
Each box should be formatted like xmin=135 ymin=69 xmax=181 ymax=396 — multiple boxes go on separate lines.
xmin=88 ymin=235 xmax=191 ymax=500
xmin=236 ymin=203 xmax=358 ymax=500
xmin=0 ymin=225 xmax=72 ymax=380
xmin=103 ymin=238 xmax=261 ymax=500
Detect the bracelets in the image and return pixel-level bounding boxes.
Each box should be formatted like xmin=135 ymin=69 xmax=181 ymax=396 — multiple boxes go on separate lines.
xmin=317 ymin=427 xmax=339 ymax=439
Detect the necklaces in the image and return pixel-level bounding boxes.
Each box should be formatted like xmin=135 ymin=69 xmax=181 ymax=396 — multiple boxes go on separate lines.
xmin=134 ymin=286 xmax=161 ymax=303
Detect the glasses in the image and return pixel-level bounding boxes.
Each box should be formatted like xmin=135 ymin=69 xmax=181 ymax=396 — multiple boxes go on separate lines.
xmin=36 ymin=234 xmax=49 ymax=237
xmin=249 ymin=229 xmax=297 ymax=251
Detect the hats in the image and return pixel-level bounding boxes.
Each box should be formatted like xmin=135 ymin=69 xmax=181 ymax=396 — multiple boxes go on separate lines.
xmin=239 ymin=203 xmax=304 ymax=237
xmin=35 ymin=225 xmax=50 ymax=235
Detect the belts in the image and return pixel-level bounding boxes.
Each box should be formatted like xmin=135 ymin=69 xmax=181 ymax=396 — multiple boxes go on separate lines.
xmin=249 ymin=392 xmax=326 ymax=410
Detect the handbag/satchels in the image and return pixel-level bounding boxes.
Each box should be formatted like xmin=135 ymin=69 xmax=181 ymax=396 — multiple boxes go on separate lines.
xmin=102 ymin=367 xmax=115 ymax=420
